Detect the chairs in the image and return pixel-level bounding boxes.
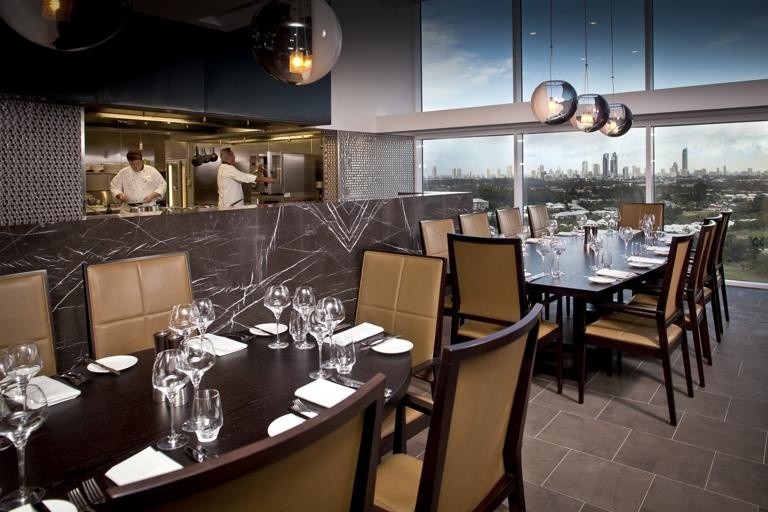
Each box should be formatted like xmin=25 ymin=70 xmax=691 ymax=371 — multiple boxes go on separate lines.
xmin=577 ymin=231 xmax=697 ymax=427
xmin=79 ymin=250 xmax=199 ymax=362
xmin=323 ymin=247 xmax=449 ymax=464
xmin=419 ymin=199 xmax=733 ymax=392
xmin=1 ymin=268 xmax=57 ymax=390
xmin=367 ymin=300 xmax=541 ymax=511
xmin=84 ymin=371 xmax=388 ymax=511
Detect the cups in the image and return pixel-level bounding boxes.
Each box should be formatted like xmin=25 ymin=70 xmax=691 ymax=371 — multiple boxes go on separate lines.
xmin=192 ymin=387 xmax=224 ymax=443
xmin=334 ymin=338 xmax=356 ymax=376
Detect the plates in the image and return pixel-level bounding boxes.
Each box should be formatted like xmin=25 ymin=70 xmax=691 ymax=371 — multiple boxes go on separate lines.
xmin=87 ymin=355 xmax=138 ymax=374
xmin=655 ymin=250 xmax=669 ymax=256
xmin=268 ymin=410 xmax=318 ymax=438
xmin=369 ymin=338 xmax=415 ymax=355
xmin=250 ymin=321 xmax=289 ymax=338
xmin=589 ymin=275 xmax=616 ymax=283
xmin=8 ymin=497 xmax=78 ymax=512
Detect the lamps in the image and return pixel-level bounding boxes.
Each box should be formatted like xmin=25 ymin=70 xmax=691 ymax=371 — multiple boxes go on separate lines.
xmin=285 ymin=2 xmax=316 ymax=78
xmin=34 ymin=1 xmax=65 ymax=20
xmin=531 ymin=0 xmax=634 ymax=138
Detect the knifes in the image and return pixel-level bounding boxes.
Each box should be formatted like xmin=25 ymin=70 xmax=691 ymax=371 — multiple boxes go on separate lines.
xmin=331 ymin=374 xmax=394 ymax=398
xmin=87 ymin=358 xmax=122 ymax=378
xmin=359 ymin=333 xmax=402 ymax=351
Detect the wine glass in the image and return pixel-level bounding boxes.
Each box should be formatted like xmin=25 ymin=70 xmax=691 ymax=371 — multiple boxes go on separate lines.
xmin=490 ymin=211 xmax=656 ymax=279
xmin=0 ymin=339 xmax=48 ymax=512
xmin=151 ymin=296 xmax=215 ymax=451
xmin=262 ymin=284 xmax=347 ymax=384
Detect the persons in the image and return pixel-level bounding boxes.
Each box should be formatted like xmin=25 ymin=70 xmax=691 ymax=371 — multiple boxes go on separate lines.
xmin=109 ymin=150 xmax=167 ymax=214
xmin=216 ymin=148 xmax=276 ymax=208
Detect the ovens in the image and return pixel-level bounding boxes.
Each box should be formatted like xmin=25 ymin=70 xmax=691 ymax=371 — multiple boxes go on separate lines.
xmin=249 ymin=152 xmax=317 ymax=195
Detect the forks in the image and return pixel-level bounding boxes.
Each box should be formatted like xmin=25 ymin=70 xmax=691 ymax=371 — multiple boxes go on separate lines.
xmin=290 ymin=398 xmax=318 ymax=419
xmin=59 ymin=368 xmax=90 ymax=387
xmin=68 ymin=477 xmax=106 ymax=512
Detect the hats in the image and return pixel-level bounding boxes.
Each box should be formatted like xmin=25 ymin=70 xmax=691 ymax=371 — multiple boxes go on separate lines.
xmin=126 ymin=149 xmax=143 ymax=162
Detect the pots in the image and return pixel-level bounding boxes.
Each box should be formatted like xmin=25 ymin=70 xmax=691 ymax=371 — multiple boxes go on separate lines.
xmin=192 ymin=145 xmax=218 ymax=168
